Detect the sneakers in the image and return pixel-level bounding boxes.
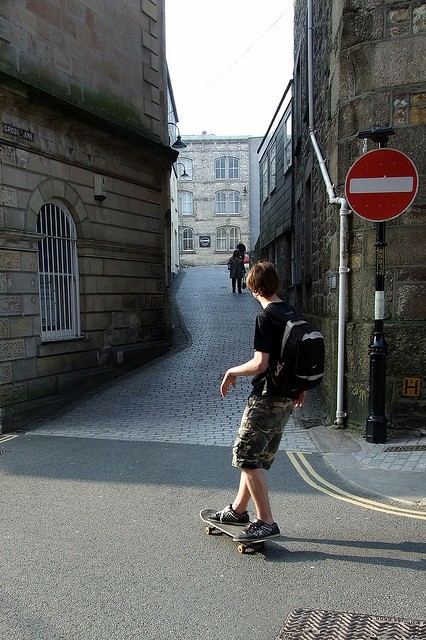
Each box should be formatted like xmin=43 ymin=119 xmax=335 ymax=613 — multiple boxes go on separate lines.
xmin=208 ymin=504 xmax=249 ymax=525
xmin=233 ymin=519 xmax=280 ymax=541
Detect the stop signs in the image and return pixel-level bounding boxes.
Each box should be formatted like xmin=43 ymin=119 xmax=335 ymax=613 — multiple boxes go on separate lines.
xmin=344 ymin=148 xmax=421 ymax=224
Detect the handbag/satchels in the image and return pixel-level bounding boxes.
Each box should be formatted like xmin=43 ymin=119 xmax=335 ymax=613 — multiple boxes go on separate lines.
xmin=241 ymin=282 xmax=246 ymax=289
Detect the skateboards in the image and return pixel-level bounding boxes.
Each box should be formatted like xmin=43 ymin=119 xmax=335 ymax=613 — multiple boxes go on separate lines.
xmin=200 ymin=508 xmax=279 ymax=554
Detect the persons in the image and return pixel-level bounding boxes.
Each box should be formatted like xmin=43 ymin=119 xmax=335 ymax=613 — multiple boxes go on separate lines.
xmin=227 ymin=250 xmax=245 ymax=294
xmin=210 ymin=260 xmax=300 ymax=543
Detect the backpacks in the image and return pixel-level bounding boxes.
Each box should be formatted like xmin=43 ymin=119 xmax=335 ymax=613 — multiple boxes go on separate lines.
xmin=263 ymin=313 xmax=325 ymax=397
